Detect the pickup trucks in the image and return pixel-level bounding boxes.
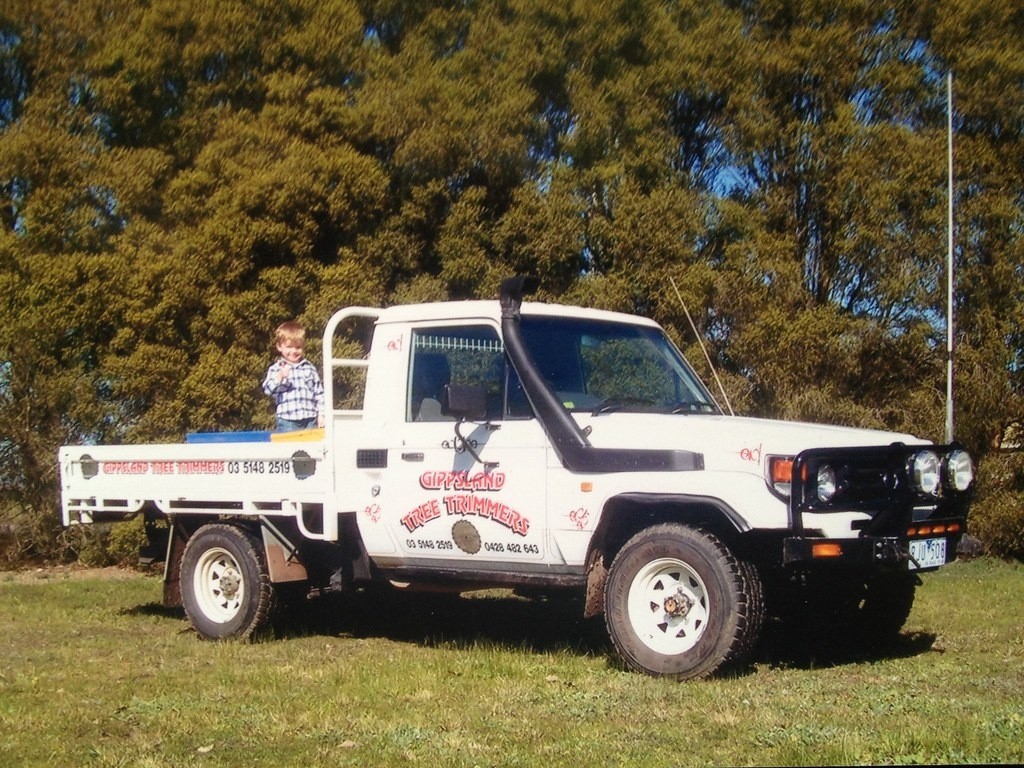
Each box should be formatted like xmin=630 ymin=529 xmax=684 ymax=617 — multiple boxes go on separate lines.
xmin=60 ymin=297 xmax=979 ymax=688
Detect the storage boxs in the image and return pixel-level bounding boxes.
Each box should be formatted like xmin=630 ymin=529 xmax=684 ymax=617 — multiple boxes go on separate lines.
xmin=185 ymin=428 xmax=325 ymax=441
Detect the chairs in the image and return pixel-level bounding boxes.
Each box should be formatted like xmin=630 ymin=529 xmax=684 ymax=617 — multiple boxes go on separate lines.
xmin=413 ymin=352 xmax=451 ymax=418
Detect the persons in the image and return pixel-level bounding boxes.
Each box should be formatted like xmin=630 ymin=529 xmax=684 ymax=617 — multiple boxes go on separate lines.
xmin=261 ymin=322 xmax=323 ymax=434
